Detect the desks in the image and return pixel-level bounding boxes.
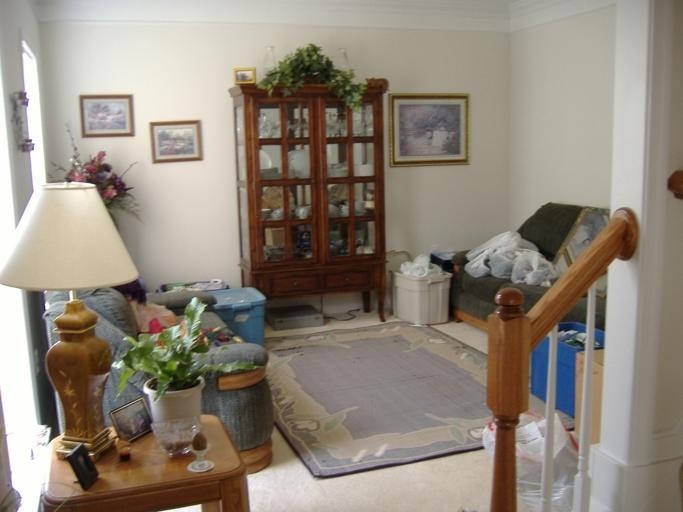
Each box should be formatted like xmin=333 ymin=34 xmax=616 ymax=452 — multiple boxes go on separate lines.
xmin=39 ymin=414 xmax=252 ymax=512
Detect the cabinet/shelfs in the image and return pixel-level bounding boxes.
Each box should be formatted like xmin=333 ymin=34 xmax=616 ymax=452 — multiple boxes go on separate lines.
xmin=228 ymin=78 xmax=391 ymax=323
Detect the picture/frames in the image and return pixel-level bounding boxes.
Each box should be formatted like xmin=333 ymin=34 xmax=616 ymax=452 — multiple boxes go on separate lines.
xmin=232 ymin=67 xmax=257 ymax=84
xmin=67 ymin=443 xmax=99 ymax=489
xmin=108 ymin=399 xmax=153 ymax=441
xmin=388 ymin=93 xmax=470 ymax=167
xmin=80 ymin=94 xmax=134 ymax=137
xmin=149 ymin=119 xmax=202 ymax=163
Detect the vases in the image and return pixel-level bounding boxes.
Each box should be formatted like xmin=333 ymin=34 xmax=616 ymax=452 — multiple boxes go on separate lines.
xmin=143 ymin=377 xmax=205 ymax=422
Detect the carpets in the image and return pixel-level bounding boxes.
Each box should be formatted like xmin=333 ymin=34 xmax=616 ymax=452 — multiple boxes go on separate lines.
xmin=271 ymin=321 xmax=488 ymax=479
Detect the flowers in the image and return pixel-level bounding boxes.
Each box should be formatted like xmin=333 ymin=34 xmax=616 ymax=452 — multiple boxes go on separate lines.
xmin=110 ymin=297 xmax=267 ymax=401
xmin=45 ymin=119 xmax=144 ymax=227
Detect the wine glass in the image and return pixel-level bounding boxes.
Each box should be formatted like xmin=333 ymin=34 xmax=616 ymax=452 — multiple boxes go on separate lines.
xmin=257 ymin=111 xmax=373 ymax=138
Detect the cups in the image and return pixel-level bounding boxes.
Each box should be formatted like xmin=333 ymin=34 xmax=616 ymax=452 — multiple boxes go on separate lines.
xmin=261 ymin=201 xmax=377 ymax=221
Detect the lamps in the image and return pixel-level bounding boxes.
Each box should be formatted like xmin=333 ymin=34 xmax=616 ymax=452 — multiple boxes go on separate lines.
xmin=0 ymin=181 xmax=138 ymax=460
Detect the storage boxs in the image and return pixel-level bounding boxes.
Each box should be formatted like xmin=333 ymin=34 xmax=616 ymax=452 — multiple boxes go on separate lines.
xmin=530 ymin=323 xmax=605 ymax=416
xmin=212 ymin=287 xmax=265 ymax=348
xmin=573 ymin=349 xmax=606 ymax=443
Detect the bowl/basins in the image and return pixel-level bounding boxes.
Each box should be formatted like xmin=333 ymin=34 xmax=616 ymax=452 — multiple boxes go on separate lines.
xmin=150 ymin=416 xmax=200 ymax=458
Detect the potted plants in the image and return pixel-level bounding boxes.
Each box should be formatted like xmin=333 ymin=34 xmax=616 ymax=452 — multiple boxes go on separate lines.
xmin=259 ymin=41 xmax=367 ymax=112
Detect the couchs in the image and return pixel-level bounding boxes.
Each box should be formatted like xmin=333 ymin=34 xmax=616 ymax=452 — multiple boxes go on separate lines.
xmin=42 ymin=288 xmax=271 ymax=476
xmin=428 ymin=201 xmax=611 ymax=333
